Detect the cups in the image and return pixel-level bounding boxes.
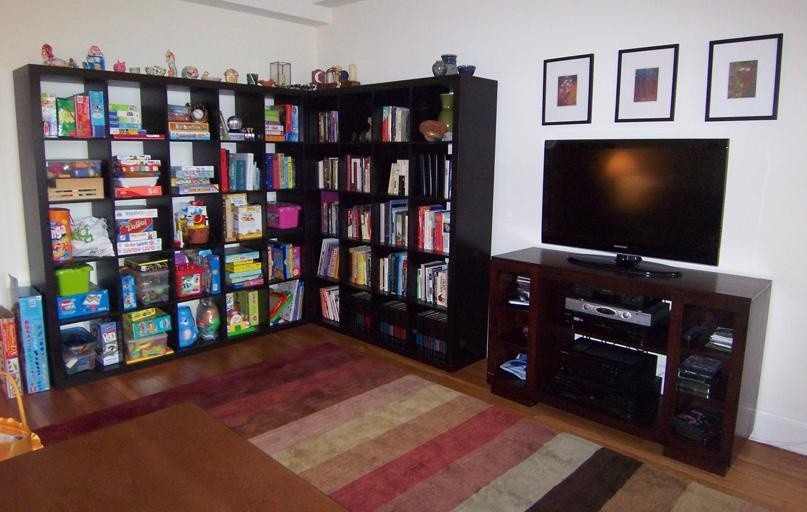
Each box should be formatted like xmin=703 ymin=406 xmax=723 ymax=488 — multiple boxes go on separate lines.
xmin=441 ymin=55 xmax=457 ymax=75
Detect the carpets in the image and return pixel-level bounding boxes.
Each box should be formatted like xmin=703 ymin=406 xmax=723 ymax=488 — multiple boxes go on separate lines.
xmin=33 ymin=338 xmax=769 ymax=510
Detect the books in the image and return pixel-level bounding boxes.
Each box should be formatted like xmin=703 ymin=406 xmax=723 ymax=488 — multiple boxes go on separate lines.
xmin=316 ymin=105 xmax=453 ymax=361
xmin=705 ymin=326 xmax=735 ymax=353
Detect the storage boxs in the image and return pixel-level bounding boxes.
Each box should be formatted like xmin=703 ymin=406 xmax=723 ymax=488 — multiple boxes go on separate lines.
xmin=0 ymin=305 xmax=24 ymax=399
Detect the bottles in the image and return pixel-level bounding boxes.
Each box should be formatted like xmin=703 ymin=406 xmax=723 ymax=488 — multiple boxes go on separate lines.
xmin=326 ymin=64 xmax=358 ymax=90
xmin=437 ymin=94 xmax=453 ymax=133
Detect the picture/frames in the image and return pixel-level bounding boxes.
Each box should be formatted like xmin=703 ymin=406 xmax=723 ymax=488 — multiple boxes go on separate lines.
xmin=541 ymin=31 xmax=784 ymax=126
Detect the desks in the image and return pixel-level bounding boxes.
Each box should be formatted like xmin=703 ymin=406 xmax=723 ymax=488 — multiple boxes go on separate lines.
xmin=0 ymin=397 xmax=380 ymax=511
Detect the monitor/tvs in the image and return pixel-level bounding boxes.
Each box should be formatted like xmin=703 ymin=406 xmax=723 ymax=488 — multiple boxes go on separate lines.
xmin=542 ymin=138 xmax=729 ymax=278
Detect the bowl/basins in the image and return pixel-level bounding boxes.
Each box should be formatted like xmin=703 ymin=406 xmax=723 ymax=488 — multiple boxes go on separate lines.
xmin=457 ymin=65 xmax=477 ymax=76
